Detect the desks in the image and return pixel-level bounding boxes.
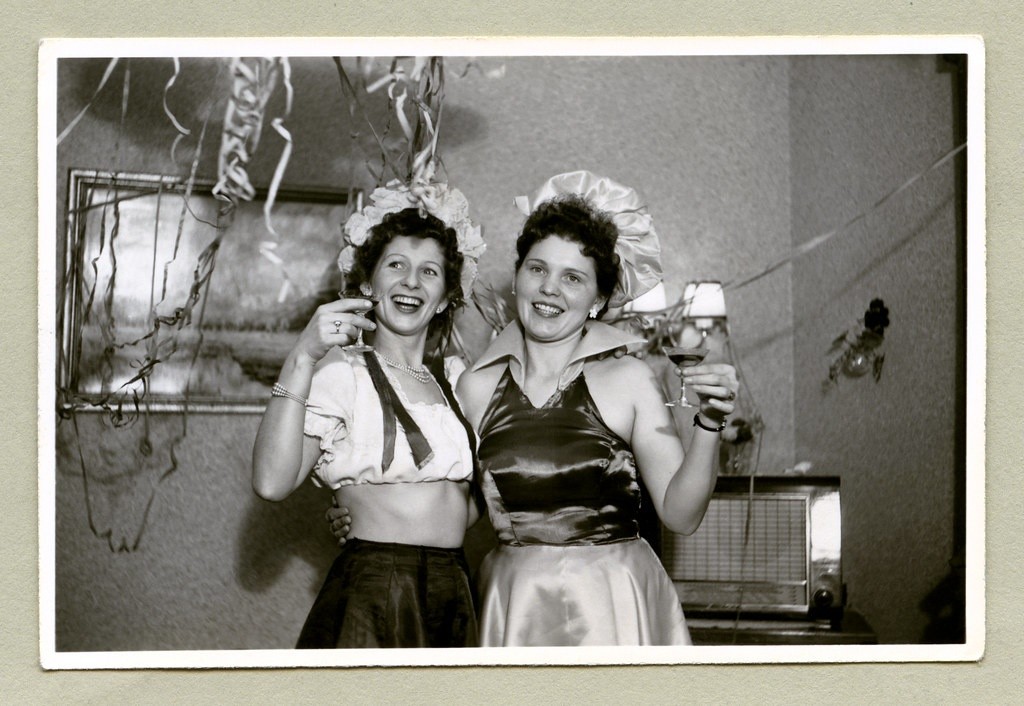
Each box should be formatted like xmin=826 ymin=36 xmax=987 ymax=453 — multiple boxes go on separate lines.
xmin=685 ymin=608 xmax=885 ymax=646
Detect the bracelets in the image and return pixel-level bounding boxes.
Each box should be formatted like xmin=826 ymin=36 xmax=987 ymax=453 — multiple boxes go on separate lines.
xmin=270 ymin=382 xmax=309 ymax=407
xmin=692 ymin=411 xmax=728 ymax=433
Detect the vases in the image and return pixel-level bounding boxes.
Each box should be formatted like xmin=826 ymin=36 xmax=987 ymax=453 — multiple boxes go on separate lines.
xmin=846 ymin=353 xmax=871 ymax=378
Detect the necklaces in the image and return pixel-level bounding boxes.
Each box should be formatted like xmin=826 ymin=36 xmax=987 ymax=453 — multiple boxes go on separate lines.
xmin=365 ymin=345 xmax=432 ymax=384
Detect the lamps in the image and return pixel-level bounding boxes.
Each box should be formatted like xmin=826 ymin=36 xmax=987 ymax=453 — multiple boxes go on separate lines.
xmin=623 ymin=280 xmax=727 ymax=356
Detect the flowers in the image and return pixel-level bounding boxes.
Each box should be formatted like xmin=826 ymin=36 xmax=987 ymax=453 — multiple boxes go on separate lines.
xmin=830 ymin=298 xmax=891 ymax=384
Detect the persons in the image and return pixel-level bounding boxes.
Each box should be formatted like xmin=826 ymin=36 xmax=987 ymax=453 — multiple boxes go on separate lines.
xmin=250 ymin=176 xmax=647 ymax=648
xmin=324 ymin=170 xmax=742 ymax=647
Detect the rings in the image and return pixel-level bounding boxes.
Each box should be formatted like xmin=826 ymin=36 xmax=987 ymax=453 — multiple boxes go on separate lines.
xmin=729 ymin=390 xmax=736 ymax=401
xmin=333 ymin=320 xmax=342 ymax=334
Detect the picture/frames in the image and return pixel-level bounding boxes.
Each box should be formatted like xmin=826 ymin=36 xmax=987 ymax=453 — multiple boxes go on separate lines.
xmin=58 ymin=166 xmax=364 ymax=413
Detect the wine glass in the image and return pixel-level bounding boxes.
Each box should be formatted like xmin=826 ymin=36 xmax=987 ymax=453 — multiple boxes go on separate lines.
xmin=338 ymin=288 xmax=384 ymax=352
xmin=655 ymin=321 xmax=713 ymax=409
